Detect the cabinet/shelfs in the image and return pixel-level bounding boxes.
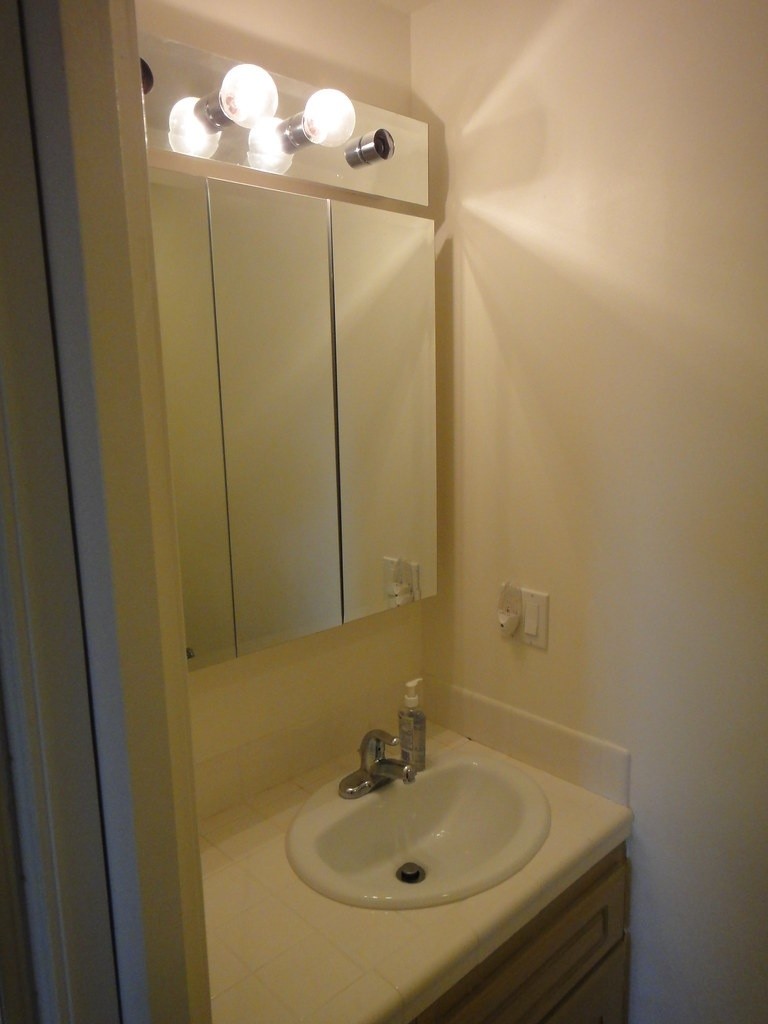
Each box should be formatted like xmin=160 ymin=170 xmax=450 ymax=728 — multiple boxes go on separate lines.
xmin=413 ymin=840 xmax=632 ymax=1024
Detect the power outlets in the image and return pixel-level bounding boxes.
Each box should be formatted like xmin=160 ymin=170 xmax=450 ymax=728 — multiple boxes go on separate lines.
xmin=495 ymin=582 xmax=549 ymax=650
xmin=383 ymin=555 xmax=421 ymax=609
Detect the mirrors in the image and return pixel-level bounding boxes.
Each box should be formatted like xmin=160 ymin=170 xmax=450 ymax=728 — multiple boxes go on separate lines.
xmin=130 ymin=29 xmax=451 ymax=677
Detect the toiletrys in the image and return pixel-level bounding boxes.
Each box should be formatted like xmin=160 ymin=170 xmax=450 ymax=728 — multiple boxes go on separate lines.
xmin=397 ymin=676 xmax=431 ymax=775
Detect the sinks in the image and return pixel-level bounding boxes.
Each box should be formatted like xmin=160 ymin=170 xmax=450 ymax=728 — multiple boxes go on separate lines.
xmin=282 ymin=749 xmax=552 ymax=912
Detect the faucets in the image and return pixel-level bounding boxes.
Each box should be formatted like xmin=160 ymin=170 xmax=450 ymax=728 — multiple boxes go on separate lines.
xmin=356 ymin=728 xmax=419 ymax=788
